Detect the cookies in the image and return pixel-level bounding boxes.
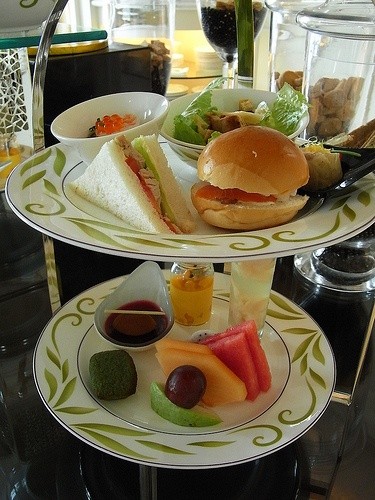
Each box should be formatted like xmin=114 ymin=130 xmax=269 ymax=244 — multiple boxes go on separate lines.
xmin=271 ymin=70 xmax=364 ymax=137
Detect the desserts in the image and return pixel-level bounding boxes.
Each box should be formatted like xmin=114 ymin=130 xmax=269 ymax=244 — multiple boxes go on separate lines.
xmin=298 ymin=142 xmax=343 ymax=188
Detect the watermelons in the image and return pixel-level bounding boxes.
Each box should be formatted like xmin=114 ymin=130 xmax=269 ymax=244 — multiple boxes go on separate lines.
xmin=206 ymin=332 xmax=260 ymax=401
xmin=198 ymin=320 xmax=272 ymax=393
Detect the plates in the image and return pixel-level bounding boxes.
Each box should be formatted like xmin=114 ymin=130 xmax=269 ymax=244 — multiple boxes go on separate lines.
xmin=93 ymin=261 xmax=175 ymax=352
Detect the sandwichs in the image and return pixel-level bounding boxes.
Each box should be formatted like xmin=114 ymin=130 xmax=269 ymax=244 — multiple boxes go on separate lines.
xmin=69 ymin=134 xmax=197 ymax=235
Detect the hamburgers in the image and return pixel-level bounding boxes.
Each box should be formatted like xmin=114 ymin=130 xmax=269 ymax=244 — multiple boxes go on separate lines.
xmin=190 ymin=125 xmax=312 ymax=230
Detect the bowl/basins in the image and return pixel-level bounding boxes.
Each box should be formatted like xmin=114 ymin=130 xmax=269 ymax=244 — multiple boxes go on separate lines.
xmin=50 ymin=91 xmax=170 ymax=166
xmin=0 ymin=0 xmax=56 ymax=34
xmin=159 ymin=88 xmax=310 ymax=169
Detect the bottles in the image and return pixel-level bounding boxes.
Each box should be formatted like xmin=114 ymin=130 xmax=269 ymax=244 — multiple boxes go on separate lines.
xmin=285 ymin=225 xmax=375 ymax=405
xmin=262 ymin=0 xmax=375 ymax=144
xmin=170 ymin=261 xmax=214 ymax=327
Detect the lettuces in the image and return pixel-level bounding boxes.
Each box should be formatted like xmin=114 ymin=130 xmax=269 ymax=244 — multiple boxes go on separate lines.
xmin=171 ymin=76 xmax=310 ymax=146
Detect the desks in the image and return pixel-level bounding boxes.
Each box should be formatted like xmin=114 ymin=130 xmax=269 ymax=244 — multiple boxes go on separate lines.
xmin=1 ymin=31 xmax=375 ymax=500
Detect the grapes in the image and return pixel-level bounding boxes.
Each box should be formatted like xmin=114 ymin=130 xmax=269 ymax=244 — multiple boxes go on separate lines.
xmin=165 ymin=365 xmax=206 ymax=409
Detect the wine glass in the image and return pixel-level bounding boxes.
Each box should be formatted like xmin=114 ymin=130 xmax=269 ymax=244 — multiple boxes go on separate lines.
xmin=196 ymin=0 xmax=268 ymax=88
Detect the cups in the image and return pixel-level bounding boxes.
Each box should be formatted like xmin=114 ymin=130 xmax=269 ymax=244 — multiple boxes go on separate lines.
xmin=66 ymin=1 xmax=176 ymax=67
xmin=226 ymin=257 xmax=277 ymax=337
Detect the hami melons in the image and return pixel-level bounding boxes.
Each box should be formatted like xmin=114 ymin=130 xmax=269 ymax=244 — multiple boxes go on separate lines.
xmin=154 ymin=340 xmax=210 ymax=355
xmin=155 ymin=349 xmax=248 ymax=408
